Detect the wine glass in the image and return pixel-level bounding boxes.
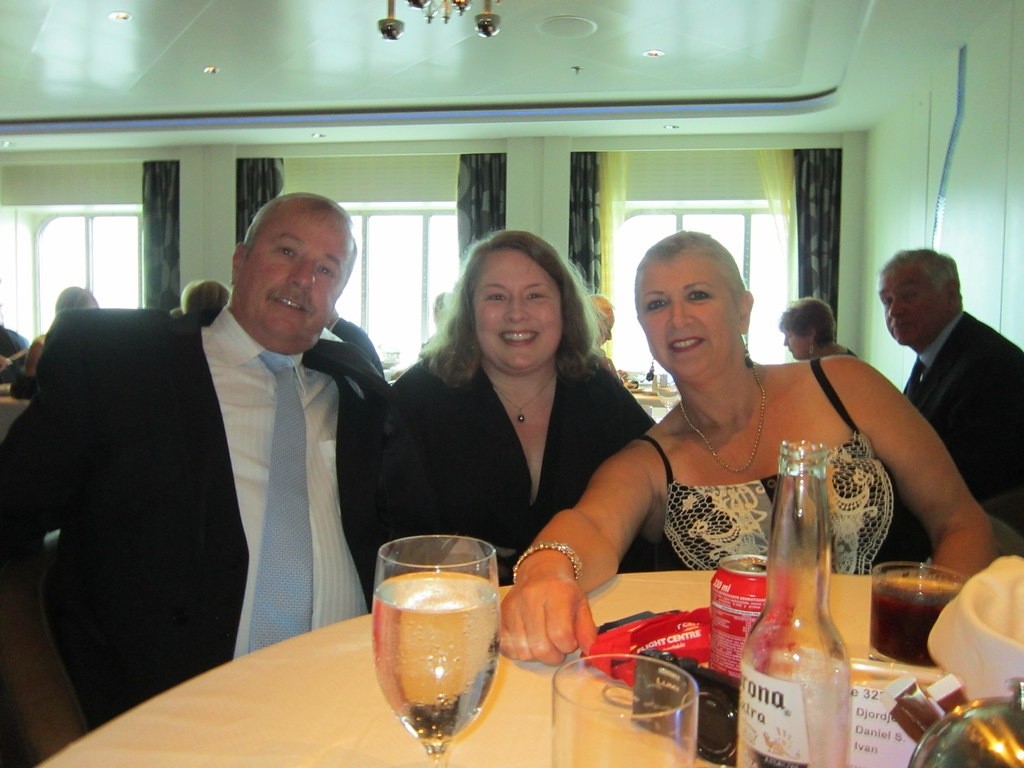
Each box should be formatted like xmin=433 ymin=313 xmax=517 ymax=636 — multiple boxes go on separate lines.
xmin=657 ymin=383 xmax=679 ymax=413
xmin=369 ymin=533 xmax=502 ymax=768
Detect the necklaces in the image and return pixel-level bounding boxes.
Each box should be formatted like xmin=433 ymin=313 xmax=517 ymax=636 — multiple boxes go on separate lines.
xmin=680 ymin=366 xmax=765 ymax=473
xmin=494 ymin=369 xmax=555 ymax=425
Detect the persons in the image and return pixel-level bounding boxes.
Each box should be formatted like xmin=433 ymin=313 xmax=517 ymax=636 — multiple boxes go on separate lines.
xmin=876 ymin=248 xmax=1024 ymax=558
xmin=391 ymin=230 xmax=656 ymax=586
xmin=779 ymin=297 xmax=857 ymax=360
xmin=386 ymin=293 xmax=625 ymax=387
xmin=503 ymin=230 xmax=992 ymax=667
xmin=0 ymin=280 xmax=229 ymax=400
xmin=0 ymin=193 xmax=435 ymax=767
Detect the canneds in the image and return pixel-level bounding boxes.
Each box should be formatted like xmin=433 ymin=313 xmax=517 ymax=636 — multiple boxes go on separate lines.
xmin=707 ymin=554 xmax=768 ymax=683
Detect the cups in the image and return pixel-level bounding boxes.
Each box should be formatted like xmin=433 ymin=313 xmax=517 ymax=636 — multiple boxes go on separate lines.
xmin=866 ymin=561 xmax=967 ymax=669
xmin=551 ymin=652 xmax=700 ymax=768
xmin=959 ymin=582 xmax=1024 ymax=700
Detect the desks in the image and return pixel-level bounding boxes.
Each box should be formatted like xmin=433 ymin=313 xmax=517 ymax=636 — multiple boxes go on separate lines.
xmin=35 ymin=568 xmax=940 ymax=768
xmin=627 ymin=381 xmax=680 ymax=423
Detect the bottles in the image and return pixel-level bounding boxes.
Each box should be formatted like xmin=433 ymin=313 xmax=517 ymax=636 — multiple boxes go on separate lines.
xmin=735 ymin=441 xmax=855 ymax=768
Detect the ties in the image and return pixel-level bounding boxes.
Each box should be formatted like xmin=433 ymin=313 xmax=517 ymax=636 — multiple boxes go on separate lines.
xmin=249 ymin=350 xmax=313 ymax=651
xmin=904 ymin=358 xmax=926 ymax=398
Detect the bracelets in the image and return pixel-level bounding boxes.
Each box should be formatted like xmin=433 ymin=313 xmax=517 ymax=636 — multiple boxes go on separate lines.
xmin=513 ymin=541 xmax=584 ymax=585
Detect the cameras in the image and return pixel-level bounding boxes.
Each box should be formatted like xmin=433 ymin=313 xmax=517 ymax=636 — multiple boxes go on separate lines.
xmin=631 ymin=649 xmax=743 ymax=766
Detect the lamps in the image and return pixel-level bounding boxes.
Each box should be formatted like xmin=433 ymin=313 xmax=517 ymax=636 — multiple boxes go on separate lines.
xmin=380 ymin=1 xmax=500 ymax=39
